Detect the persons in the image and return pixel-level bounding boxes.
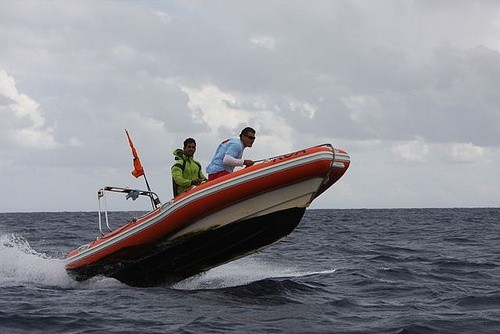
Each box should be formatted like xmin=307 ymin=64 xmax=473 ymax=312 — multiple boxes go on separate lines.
xmin=170 ymin=137 xmax=206 ymax=198
xmin=207 ymin=125 xmax=257 ymax=179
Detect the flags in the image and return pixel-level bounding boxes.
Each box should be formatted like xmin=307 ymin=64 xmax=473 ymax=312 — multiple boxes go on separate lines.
xmin=125 ymin=129 xmax=145 ymax=178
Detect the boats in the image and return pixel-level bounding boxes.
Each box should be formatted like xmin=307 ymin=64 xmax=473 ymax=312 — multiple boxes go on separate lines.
xmin=64 ymin=127 xmax=352 ymax=290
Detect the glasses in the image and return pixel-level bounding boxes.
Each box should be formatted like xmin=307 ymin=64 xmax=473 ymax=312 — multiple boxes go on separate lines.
xmin=244 ymin=135 xmax=255 ymax=140
